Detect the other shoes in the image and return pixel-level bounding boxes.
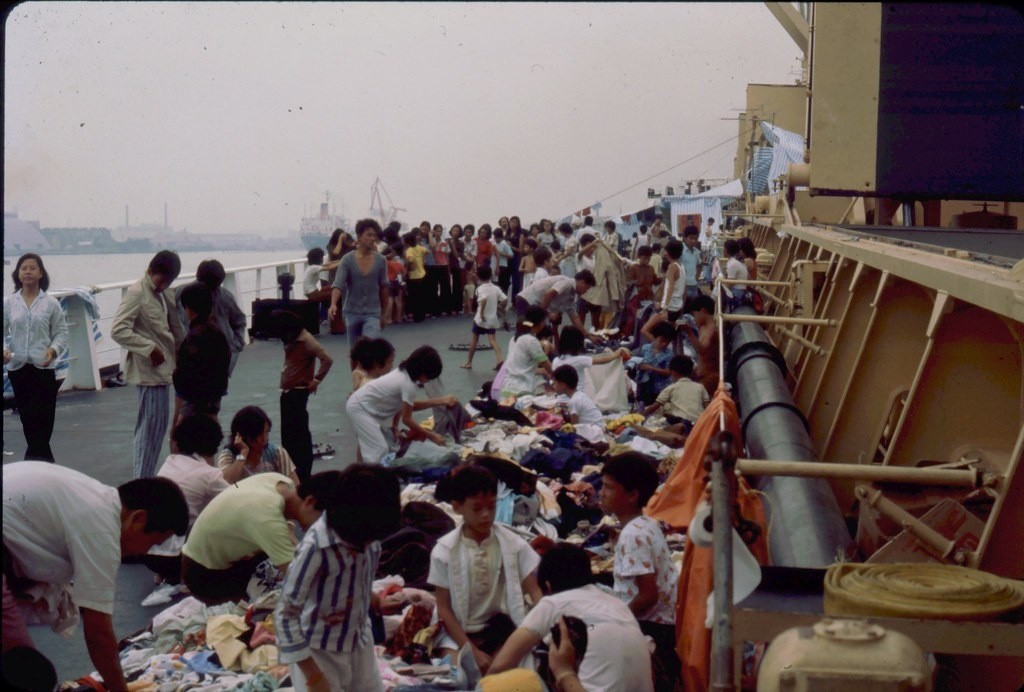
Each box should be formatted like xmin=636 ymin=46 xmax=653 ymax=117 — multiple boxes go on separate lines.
xmin=410 ymin=309 xmax=464 ymax=322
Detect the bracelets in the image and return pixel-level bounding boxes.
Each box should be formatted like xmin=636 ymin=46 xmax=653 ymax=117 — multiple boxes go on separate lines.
xmin=557 ymin=672 xmax=577 ymax=689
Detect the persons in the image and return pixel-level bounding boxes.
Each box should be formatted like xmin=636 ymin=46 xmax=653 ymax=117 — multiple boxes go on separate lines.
xmin=174 ymin=259 xmax=247 ymax=379
xmin=0 ymin=460 xmax=190 ymax=692
xmin=170 ymin=283 xmax=231 ymax=466
xmin=144 ymin=215 xmax=757 ymax=678
xmin=2 ymin=253 xmax=69 ymax=464
xmin=271 ymin=462 xmax=400 ymax=692
xmin=110 ymin=250 xmax=187 ymax=480
xmin=548 ymin=616 xmax=586 ymax=692
